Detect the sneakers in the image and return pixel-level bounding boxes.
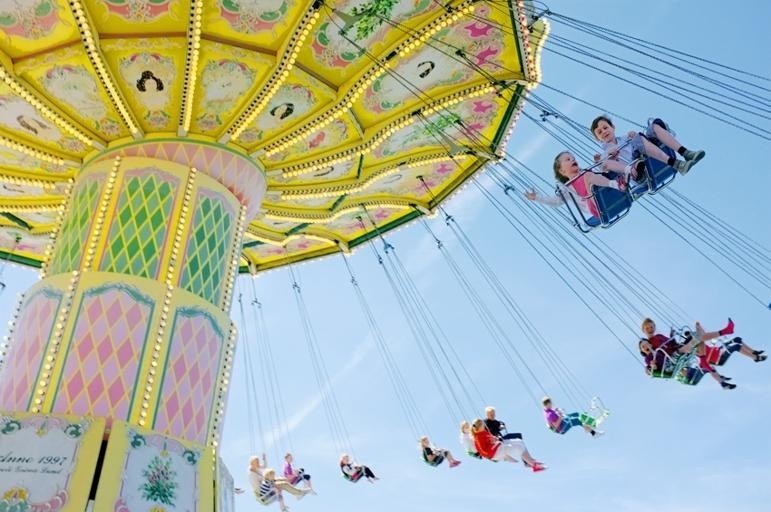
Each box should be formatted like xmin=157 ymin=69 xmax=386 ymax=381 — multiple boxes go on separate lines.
xmin=697 ymin=316 xmax=767 ymax=390
xmin=280 ymin=487 xmax=318 ymax=512
xmin=678 ymin=150 xmax=705 ymax=176
xmin=508 ymin=460 xmax=547 ymax=473
xmin=447 ymin=460 xmax=460 ymax=468
xmin=592 ymin=430 xmax=605 ymax=439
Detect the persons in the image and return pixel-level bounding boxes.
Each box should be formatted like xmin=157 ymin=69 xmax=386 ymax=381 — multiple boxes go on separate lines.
xmin=409 ymin=61 xmax=436 ymax=83
xmin=639 ymin=317 xmax=735 ymax=373
xmin=470 ymin=418 xmax=549 ymax=473
xmin=339 ymin=454 xmax=380 ymax=483
xmin=418 ymin=434 xmax=463 ymax=468
xmin=639 ymin=317 xmax=738 ymax=388
xmin=459 ymin=420 xmax=484 ymax=457
xmin=135 ymin=67 xmax=166 ymax=111
xmin=482 ymin=405 xmax=537 ymax=468
xmin=259 ymin=468 xmax=291 ymax=511
xmin=590 ymin=114 xmax=708 ymax=184
xmin=542 ymin=396 xmax=606 ymax=439
xmin=250 ymin=455 xmax=312 ymax=499
xmin=284 ymin=451 xmax=318 ymax=494
xmin=522 ymin=151 xmax=648 ymax=219
xmin=678 ymin=330 xmax=769 ymax=362
xmin=235 ymin=487 xmax=245 ymax=495
xmin=254 ymin=100 xmax=294 ymax=130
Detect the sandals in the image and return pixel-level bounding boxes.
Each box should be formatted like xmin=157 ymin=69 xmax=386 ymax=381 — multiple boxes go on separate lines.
xmin=618 ymin=160 xmax=646 ymax=193
